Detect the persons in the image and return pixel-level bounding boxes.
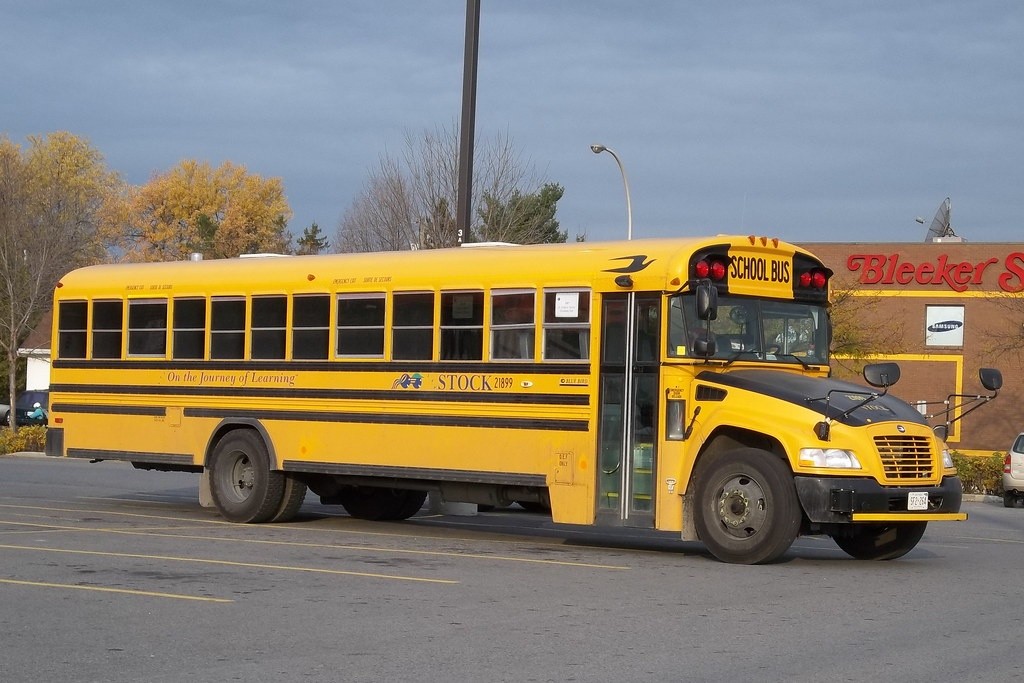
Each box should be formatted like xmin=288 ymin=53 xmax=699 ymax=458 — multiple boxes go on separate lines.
xmin=684 ymin=304 xmax=718 ymax=352
xmin=30 ymin=402 xmax=43 ymax=420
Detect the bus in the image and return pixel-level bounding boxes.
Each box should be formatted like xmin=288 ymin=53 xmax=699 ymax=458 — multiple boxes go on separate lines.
xmin=45 ymin=234 xmax=1003 ymax=566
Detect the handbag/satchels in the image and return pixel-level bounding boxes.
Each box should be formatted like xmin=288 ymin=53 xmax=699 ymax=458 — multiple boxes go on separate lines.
xmin=33 ymin=413 xmax=48 ymax=425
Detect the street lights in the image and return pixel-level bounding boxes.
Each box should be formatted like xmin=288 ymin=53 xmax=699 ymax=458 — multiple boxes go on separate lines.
xmin=590 ymin=144 xmax=631 ymax=241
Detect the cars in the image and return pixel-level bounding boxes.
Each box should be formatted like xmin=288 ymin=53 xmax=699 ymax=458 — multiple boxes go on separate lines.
xmin=4 ymin=389 xmax=49 ymax=425
xmin=1002 ymin=433 xmax=1024 ymax=508
